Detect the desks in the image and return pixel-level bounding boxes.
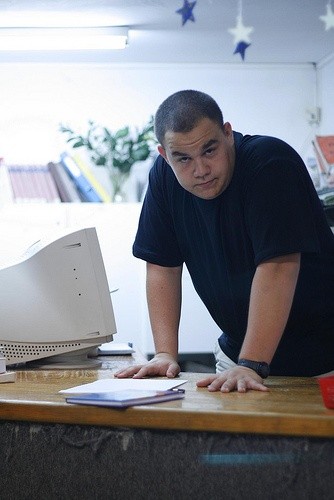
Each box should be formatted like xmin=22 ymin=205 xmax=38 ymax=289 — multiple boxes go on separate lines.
xmin=0 ymin=354 xmax=334 ymax=500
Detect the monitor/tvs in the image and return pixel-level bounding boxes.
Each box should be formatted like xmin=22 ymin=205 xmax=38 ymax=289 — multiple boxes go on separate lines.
xmin=0 ymin=227 xmax=117 ymax=365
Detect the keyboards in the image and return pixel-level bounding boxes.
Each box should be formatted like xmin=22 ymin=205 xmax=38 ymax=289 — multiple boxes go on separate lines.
xmin=98 ymin=342 xmax=136 ymax=354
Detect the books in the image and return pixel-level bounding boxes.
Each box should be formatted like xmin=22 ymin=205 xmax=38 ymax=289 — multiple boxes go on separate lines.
xmin=311 ymin=135 xmax=334 ymax=205
xmin=97 ymin=341 xmax=136 ymax=356
xmin=66 ymin=389 xmax=186 ymax=407
xmin=6 ymin=150 xmax=111 ymax=204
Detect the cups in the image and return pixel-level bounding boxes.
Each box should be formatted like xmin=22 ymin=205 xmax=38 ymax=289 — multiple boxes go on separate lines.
xmin=319 ymin=377 xmax=334 ymax=409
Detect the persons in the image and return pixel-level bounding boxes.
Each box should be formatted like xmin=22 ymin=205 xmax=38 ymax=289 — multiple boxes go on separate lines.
xmin=113 ymin=89 xmax=334 ymax=393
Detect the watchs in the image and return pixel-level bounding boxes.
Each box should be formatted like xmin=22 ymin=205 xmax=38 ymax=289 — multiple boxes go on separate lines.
xmin=238 ymin=359 xmax=270 ymax=379
